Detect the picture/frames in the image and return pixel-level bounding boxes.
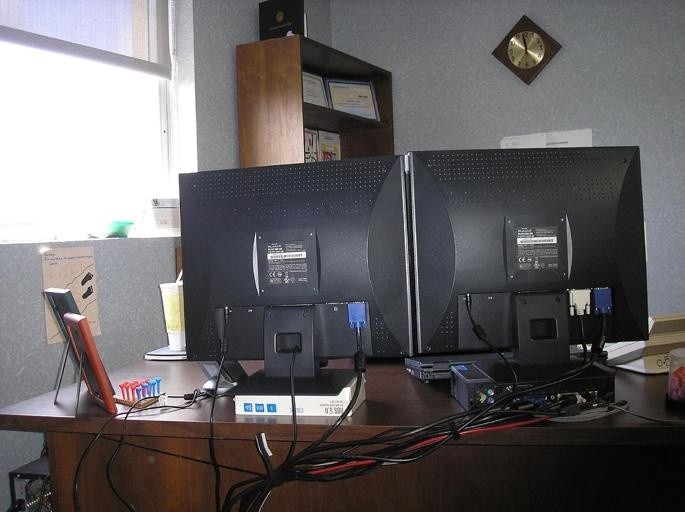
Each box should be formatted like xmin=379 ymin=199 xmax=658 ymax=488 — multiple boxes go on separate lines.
xmin=302 ymin=70 xmax=328 ymax=107
xmin=324 ymin=79 xmax=381 ymax=122
xmin=63 ymin=313 xmax=118 ymax=414
xmin=44 ymin=287 xmax=115 ymax=396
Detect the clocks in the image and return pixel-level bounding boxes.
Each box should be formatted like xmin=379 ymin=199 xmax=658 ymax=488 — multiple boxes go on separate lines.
xmin=491 ymin=14 xmax=561 ymax=84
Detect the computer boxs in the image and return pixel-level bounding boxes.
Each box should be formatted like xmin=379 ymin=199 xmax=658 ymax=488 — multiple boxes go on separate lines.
xmin=450 ymin=355 xmax=615 ymax=413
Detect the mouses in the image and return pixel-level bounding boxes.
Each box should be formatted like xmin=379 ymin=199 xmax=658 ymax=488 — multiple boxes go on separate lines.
xmin=203 ymin=378 xmax=234 ymax=394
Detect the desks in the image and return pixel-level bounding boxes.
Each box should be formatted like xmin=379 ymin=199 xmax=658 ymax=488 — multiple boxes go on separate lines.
xmin=0 ymin=353 xmax=685 ymax=512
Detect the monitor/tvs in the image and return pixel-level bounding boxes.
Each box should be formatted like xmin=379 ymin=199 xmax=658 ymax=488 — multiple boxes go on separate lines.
xmin=179 ymin=154 xmax=412 ymax=398
xmin=408 ymin=146 xmax=649 ymax=382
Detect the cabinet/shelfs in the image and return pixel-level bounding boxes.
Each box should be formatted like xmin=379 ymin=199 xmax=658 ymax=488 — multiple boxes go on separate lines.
xmin=235 ymin=36 xmax=395 ymax=168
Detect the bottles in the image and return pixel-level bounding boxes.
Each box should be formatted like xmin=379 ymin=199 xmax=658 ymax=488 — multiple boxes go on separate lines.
xmin=667 ymin=348 xmax=684 ymax=401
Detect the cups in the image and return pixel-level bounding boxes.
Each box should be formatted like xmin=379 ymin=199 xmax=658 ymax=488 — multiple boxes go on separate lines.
xmin=159 ymin=282 xmax=185 ymax=350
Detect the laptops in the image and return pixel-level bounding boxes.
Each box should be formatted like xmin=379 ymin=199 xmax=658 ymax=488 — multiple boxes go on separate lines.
xmin=404 ymin=350 xmax=609 ymax=385
